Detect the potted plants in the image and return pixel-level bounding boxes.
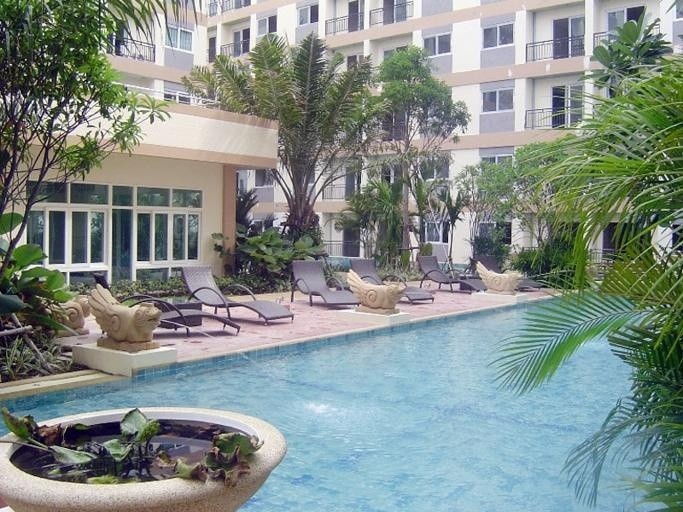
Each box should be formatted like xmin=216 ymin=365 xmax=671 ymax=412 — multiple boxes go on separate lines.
xmin=0 ymin=405 xmax=288 ymax=512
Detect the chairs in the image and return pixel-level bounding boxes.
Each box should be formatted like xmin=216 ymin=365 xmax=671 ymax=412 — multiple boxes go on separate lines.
xmin=92 ymin=272 xmax=240 ymax=337
xmin=349 ymin=257 xmax=435 ymax=305
xmin=181 ymin=266 xmax=295 ymax=326
xmin=479 ymin=255 xmax=541 ymax=293
xmin=417 ymin=255 xmax=488 ymax=295
xmin=289 ymin=259 xmax=362 ymax=310
